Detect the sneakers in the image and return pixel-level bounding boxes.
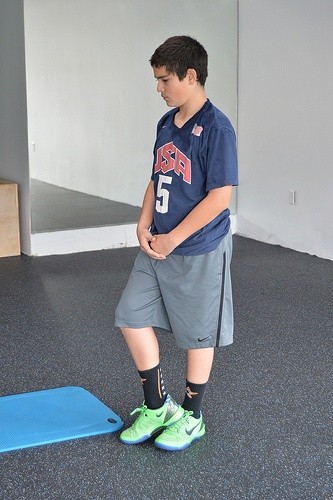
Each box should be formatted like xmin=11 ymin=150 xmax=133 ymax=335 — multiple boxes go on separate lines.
xmin=119 ymin=392 xmax=186 ymax=445
xmin=154 ymin=412 xmax=207 ymax=451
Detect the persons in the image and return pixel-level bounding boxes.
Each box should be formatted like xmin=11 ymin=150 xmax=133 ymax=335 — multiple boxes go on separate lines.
xmin=113 ymin=35 xmax=238 ymax=452
